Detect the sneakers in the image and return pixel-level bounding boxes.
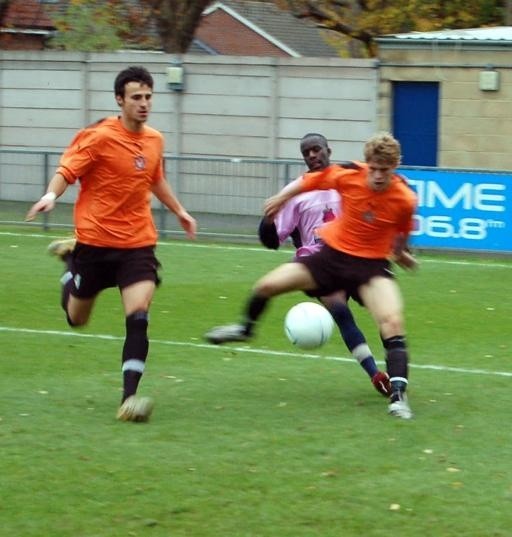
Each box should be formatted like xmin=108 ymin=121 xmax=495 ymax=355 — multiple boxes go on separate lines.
xmin=47 ymin=239 xmax=76 ymax=255
xmin=372 ymin=371 xmax=412 ymax=418
xmin=205 ymin=325 xmax=246 ymax=343
xmin=117 ymin=396 xmax=152 ymax=421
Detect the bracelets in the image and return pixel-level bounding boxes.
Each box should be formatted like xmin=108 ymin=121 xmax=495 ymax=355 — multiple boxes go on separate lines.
xmin=39 ymin=191 xmax=58 ymax=202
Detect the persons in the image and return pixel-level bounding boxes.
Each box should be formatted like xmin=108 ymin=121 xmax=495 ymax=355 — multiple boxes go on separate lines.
xmin=258 ymin=135 xmax=394 ymax=398
xmin=203 ymin=130 xmax=420 ymax=420
xmin=26 ymin=63 xmax=198 ymax=424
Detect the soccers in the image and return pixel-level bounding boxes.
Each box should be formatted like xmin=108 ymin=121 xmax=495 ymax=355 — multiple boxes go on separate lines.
xmin=285 ymin=302 xmax=334 ymax=349
